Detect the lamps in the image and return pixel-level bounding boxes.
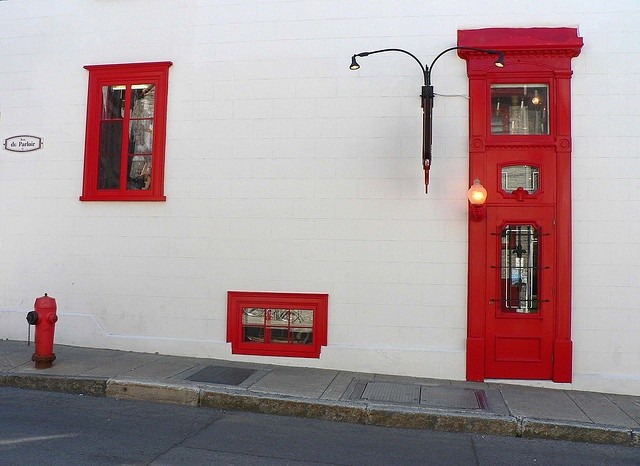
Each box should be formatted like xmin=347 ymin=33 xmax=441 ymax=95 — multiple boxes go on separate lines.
xmin=533 ymin=91 xmax=540 ymax=107
xmin=349 ymin=46 xmax=504 ymax=195
xmin=466 ymin=177 xmax=486 ymax=222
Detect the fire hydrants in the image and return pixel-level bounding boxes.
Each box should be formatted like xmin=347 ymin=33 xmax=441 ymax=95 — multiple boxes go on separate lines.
xmin=26 ymin=293 xmax=58 ymax=369
